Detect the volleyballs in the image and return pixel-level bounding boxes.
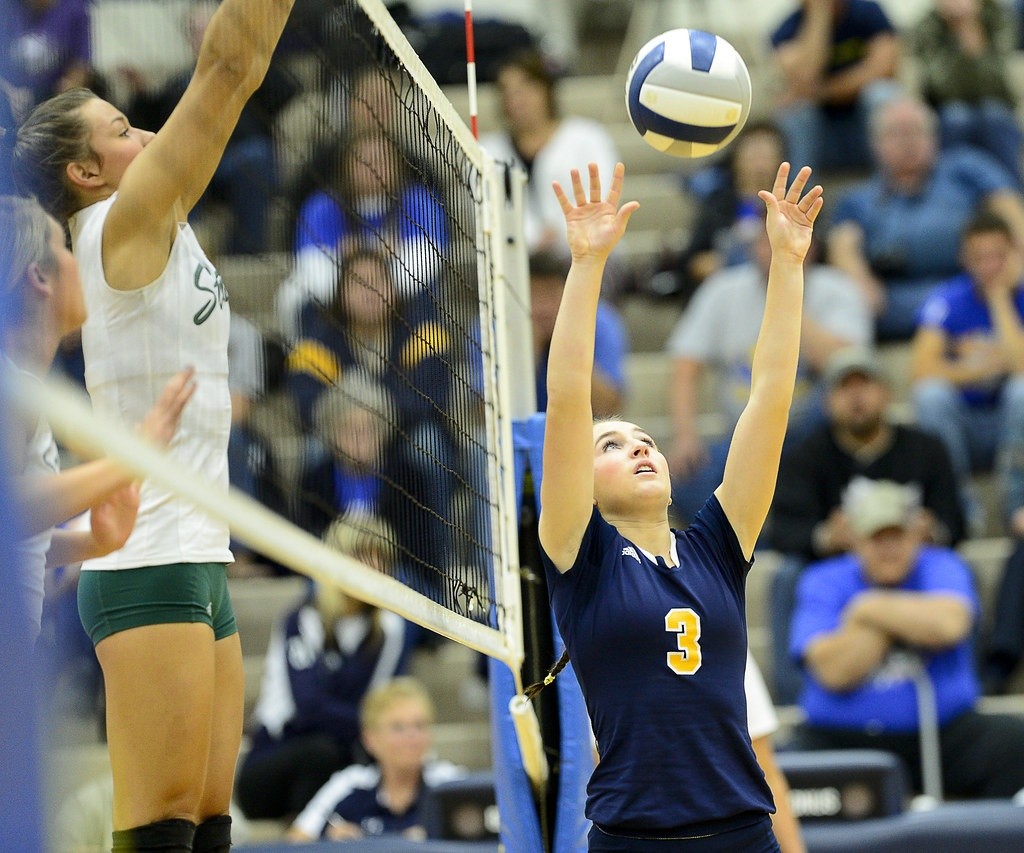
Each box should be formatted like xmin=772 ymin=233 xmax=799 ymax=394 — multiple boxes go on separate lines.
xmin=624 ymin=28 xmax=751 ymax=158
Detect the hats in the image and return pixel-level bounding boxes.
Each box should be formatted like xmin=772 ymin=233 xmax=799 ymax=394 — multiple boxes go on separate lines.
xmin=824 ymin=346 xmax=884 ymax=389
xmin=842 ymin=479 xmax=921 ymax=540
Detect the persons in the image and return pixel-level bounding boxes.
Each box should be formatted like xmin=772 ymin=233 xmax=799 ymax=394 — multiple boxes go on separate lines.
xmin=0 ymin=0 xmax=632 ymax=681
xmin=231 ymin=512 xmax=418 ymax=818
xmin=784 ymin=475 xmax=1024 ymax=816
xmin=766 ymin=351 xmax=978 ymax=659
xmin=13 ymin=0 xmax=299 ymax=853
xmin=657 ymin=2 xmax=1024 ymax=522
xmin=286 ymin=679 xmax=483 ymax=851
xmin=985 ymin=460 xmax=1024 ymax=699
xmin=517 ymin=157 xmax=831 ymax=853
xmin=281 ymin=376 xmax=453 ymax=652
xmin=0 ymin=194 xmax=199 ymax=851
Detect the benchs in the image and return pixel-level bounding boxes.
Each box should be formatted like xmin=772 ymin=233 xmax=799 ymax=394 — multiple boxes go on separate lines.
xmin=46 ymin=38 xmax=1024 ymax=851
